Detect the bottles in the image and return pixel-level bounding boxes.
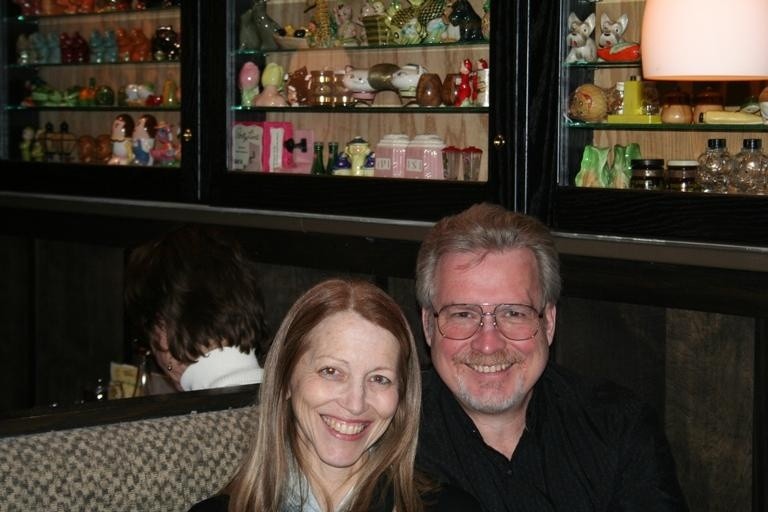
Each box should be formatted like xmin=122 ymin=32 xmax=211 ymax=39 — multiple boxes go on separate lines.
xmin=624 ymin=84 xmax=768 ymax=196
xmin=133 ymin=351 xmax=151 ymax=397
xmin=310 ymin=134 xmax=483 ymax=181
xmin=95 ymin=377 xmax=105 ymax=401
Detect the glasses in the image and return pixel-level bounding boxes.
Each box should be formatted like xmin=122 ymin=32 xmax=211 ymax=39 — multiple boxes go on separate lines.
xmin=433 ymin=304 xmax=545 ymax=341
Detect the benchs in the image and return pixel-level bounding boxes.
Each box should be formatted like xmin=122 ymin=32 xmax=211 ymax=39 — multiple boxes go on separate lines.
xmin=0 ymin=384 xmax=259 ymax=512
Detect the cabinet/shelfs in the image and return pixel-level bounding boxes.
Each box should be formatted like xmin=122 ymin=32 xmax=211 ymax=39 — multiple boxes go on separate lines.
xmin=523 ymin=0 xmax=768 ymax=254
xmin=201 ymin=0 xmax=518 ymax=223
xmin=1 ymin=0 xmax=200 ymax=201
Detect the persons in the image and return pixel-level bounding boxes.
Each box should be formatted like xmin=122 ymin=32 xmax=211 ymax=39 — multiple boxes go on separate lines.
xmin=191 ymin=280 xmax=481 ymax=512
xmin=105 ymin=114 xmax=135 ymax=165
xmin=127 ymin=234 xmax=266 ymax=396
xmin=416 ymin=203 xmax=689 ymax=510
xmin=133 ymin=115 xmax=157 ymax=166
